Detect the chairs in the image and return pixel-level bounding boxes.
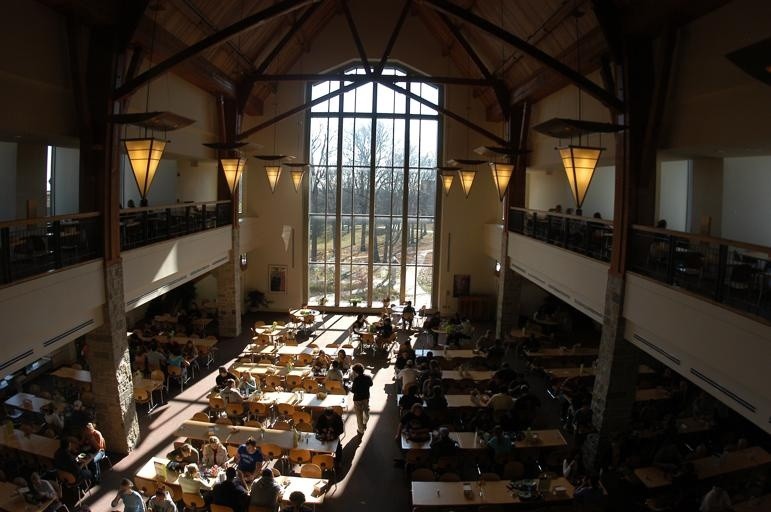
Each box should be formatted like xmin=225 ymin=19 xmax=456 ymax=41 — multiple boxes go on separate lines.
xmin=522 ymin=202 xmax=769 ymax=325
xmin=0 ymin=304 xmax=389 ymax=511
xmin=0 ymin=202 xmax=236 ymax=290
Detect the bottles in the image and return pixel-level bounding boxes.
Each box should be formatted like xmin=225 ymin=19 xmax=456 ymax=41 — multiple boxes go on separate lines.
xmin=462 ymin=483 xmax=471 ymax=500
xmin=477 ymin=474 xmax=490 ymax=499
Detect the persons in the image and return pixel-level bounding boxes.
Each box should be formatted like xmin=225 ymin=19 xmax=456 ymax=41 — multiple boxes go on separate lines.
xmin=128 ymin=301 xmax=214 ymax=384
xmin=314 ymin=349 xmax=351 ymax=389
xmin=343 ymin=363 xmax=374 ymax=436
xmin=693 ymin=392 xmax=707 ymax=414
xmin=353 ymin=312 xmax=393 ymax=352
xmin=427 ymin=312 xmax=461 ymax=345
xmin=656 ymin=219 xmax=668 ymax=229
xmin=419 ymin=305 xmax=427 ymax=317
xmin=30 ymin=423 xmax=105 ymax=510
xmin=207 ymin=367 xmax=257 ymax=414
xmin=588 ymin=211 xmax=605 ymax=250
xmin=402 ymin=301 xmax=416 ymax=332
xmin=477 ymin=329 xmax=496 ymax=353
xmin=10 ymin=385 xmax=96 ymax=440
xmin=111 ymin=436 xmax=306 ymax=511
xmin=560 ymin=374 xmax=603 ymax=496
xmin=487 ymin=425 xmax=511 ymax=466
xmin=640 ymin=398 xmax=662 ymax=427
xmin=388 ymin=304 xmax=400 ymax=328
xmin=395 ymin=340 xmax=460 ymax=464
xmin=566 ymin=207 xmax=575 ymax=216
xmin=127 ymin=200 xmax=137 ymax=220
xmin=546 ymin=205 xmax=563 ymax=236
xmin=471 ymin=374 xmax=542 ymax=423
xmin=700 ymin=482 xmax=731 ymax=509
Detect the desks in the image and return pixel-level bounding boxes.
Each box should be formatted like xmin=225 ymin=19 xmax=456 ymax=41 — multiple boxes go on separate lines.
xmin=396 ymin=204 xmax=769 ymax=510
xmin=396 ymin=307 xmax=752 ymax=509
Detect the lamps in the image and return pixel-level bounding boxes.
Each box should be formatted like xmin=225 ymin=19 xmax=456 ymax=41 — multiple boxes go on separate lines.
xmin=201 ymin=1 xmax=313 ymax=200
xmin=110 ymin=1 xmax=197 ymax=206
xmin=531 ymin=1 xmax=631 ymax=216
xmin=432 ymin=1 xmax=537 ymax=203
